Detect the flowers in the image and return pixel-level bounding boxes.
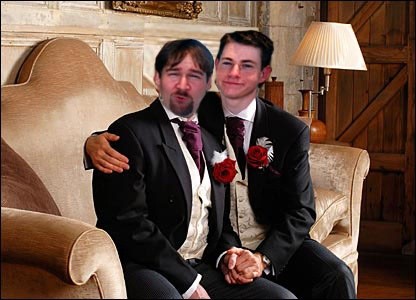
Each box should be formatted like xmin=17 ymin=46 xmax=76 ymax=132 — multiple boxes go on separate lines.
xmin=245 ymin=137 xmax=274 ymax=169
xmin=211 ymin=149 xmax=238 ymax=184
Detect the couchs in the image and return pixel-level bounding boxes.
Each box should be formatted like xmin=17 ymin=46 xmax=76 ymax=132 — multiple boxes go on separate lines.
xmin=0 ymin=37 xmax=371 ymax=299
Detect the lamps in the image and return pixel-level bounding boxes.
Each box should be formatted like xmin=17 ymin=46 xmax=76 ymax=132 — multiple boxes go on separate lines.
xmin=288 ymin=22 xmax=368 ymax=119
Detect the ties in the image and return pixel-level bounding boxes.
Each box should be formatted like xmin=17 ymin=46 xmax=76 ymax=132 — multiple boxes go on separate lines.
xmin=173 ymin=117 xmax=205 ymax=178
xmin=225 ymin=114 xmax=247 ymax=179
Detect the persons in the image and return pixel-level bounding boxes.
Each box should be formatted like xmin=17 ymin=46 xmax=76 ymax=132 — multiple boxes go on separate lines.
xmin=91 ymin=37 xmax=298 ymax=300
xmin=82 ymin=30 xmax=357 ymax=300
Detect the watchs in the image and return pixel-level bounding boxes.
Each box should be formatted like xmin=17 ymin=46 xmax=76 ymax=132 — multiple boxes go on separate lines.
xmin=261 ymin=254 xmax=271 ymax=269
